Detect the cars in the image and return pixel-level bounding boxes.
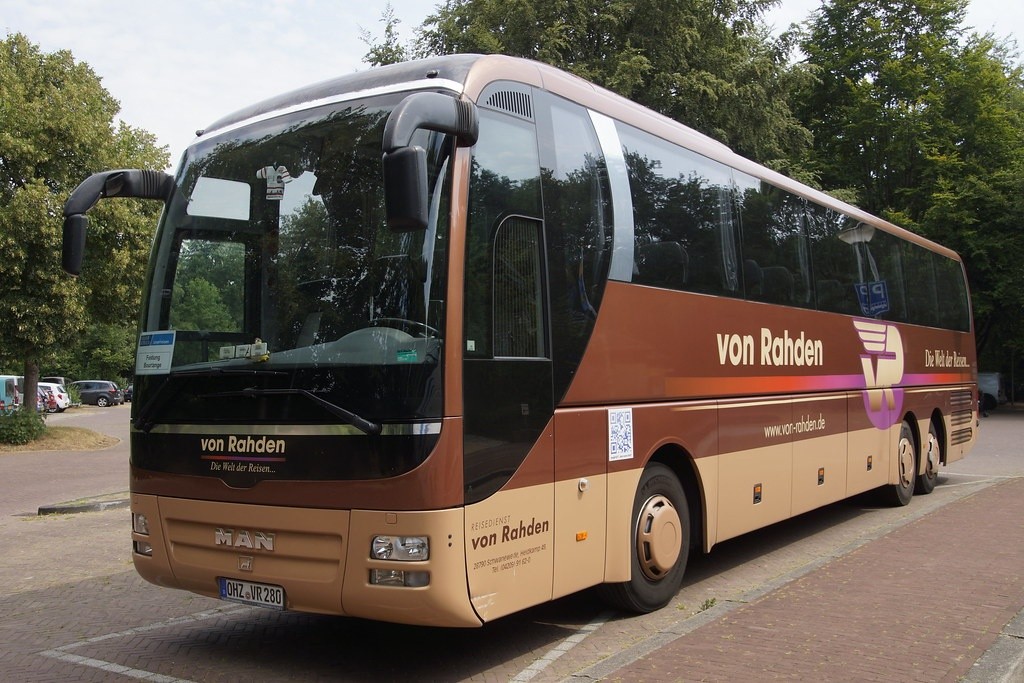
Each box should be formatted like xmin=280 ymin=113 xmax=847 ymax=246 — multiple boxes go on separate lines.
xmin=976 ymin=365 xmax=1008 ymax=417
xmin=0 ymin=375 xmax=72 ymax=416
xmin=123 ymin=385 xmax=133 ymax=402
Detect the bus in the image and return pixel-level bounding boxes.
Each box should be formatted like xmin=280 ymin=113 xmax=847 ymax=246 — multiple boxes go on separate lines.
xmin=58 ymin=53 xmax=980 ymax=629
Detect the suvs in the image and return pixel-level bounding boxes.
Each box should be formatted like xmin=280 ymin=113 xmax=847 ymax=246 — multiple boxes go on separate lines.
xmin=70 ymin=379 xmax=122 ymax=407
